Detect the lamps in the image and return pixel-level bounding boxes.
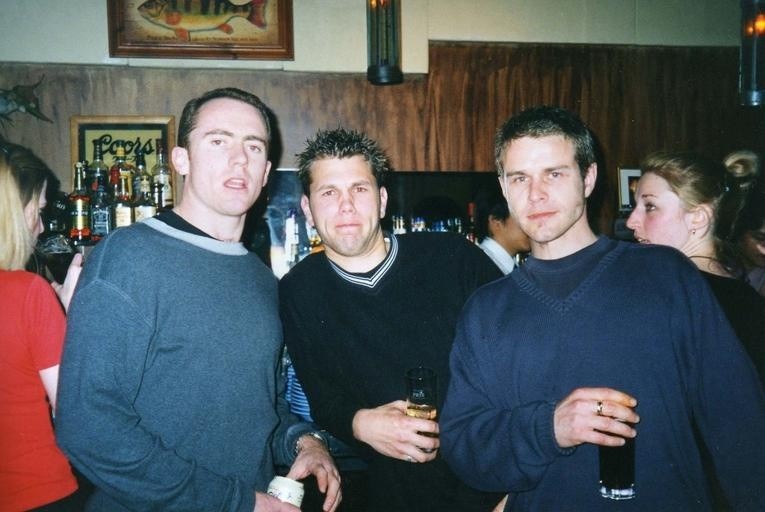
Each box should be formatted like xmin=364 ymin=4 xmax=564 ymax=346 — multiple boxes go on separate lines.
xmin=366 ymin=0 xmax=403 ymax=88
xmin=737 ymin=1 xmax=765 ymax=109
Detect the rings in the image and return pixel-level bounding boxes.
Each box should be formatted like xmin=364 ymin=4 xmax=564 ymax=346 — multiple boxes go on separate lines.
xmin=595 ymin=399 xmax=604 ymax=416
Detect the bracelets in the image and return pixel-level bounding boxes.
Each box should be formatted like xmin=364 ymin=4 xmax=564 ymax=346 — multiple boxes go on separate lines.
xmin=294 ymin=431 xmax=325 ymax=451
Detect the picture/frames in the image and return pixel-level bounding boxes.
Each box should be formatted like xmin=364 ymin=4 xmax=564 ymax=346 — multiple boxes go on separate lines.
xmin=69 ymin=113 xmax=178 ymax=208
xmin=106 ymin=0 xmax=295 ymax=61
xmin=616 ymin=164 xmax=643 ymax=214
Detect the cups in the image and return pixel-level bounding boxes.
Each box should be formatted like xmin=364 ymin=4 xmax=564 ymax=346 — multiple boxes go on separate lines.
xmin=598 ymin=410 xmax=637 ymax=499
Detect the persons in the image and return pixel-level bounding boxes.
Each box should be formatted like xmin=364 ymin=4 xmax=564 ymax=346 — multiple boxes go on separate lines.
xmin=624 ymin=150 xmax=765 ymax=370
xmin=433 ymin=103 xmax=765 ymax=510
xmin=53 ymin=87 xmax=344 ymax=512
xmin=278 ymin=130 xmax=509 ymax=512
xmin=474 ymin=180 xmax=530 ymax=274
xmin=0 ymin=139 xmax=82 ymax=512
xmin=734 ymin=207 xmax=765 ymax=289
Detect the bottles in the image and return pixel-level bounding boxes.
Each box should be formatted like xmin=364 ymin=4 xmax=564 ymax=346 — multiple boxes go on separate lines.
xmin=282 ymin=203 xmax=532 ymax=272
xmin=56 ymin=138 xmax=174 ymax=245
xmin=406 ymin=368 xmax=438 ymax=454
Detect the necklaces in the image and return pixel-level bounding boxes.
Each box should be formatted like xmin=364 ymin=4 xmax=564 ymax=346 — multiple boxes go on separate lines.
xmin=687 ymin=251 xmax=727 ymax=265
